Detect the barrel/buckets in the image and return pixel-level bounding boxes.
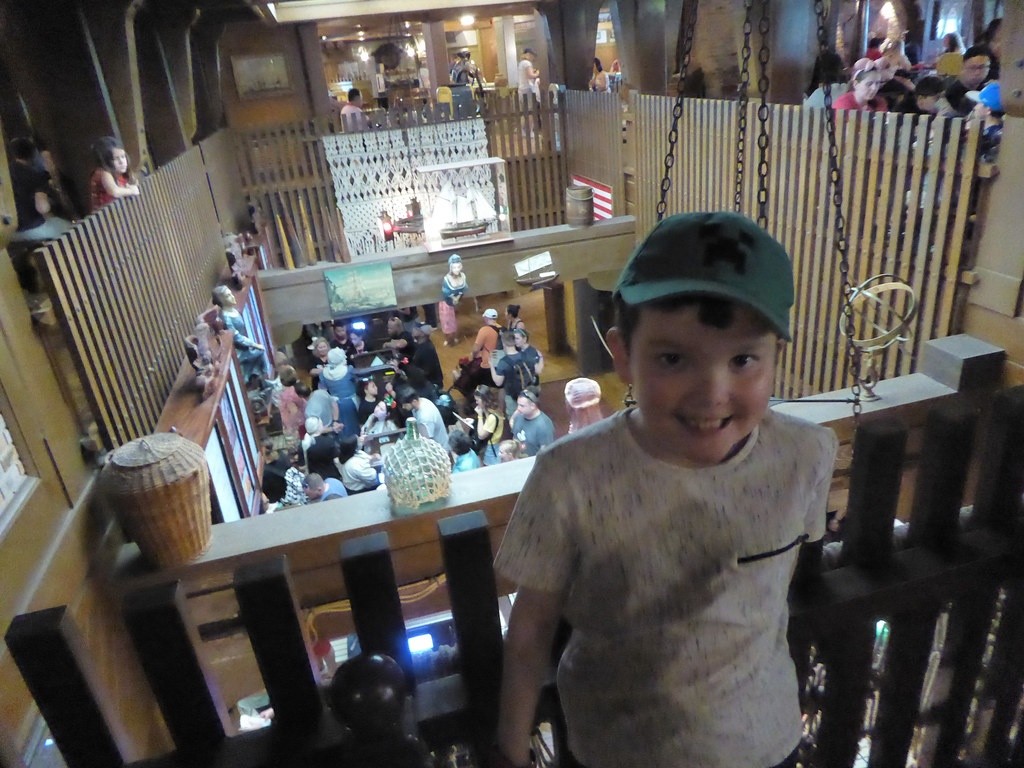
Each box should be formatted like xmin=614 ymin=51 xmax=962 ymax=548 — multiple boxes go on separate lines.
xmin=566 ymin=186 xmax=594 ymax=228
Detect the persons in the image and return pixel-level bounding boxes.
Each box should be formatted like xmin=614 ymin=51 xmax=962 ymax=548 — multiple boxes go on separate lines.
xmin=589 ymin=54 xmax=619 ymax=93
xmin=804 ymin=18 xmax=1002 ymax=216
xmin=7 ymin=137 xmax=72 ymax=225
xmin=440 ymin=254 xmax=468 ymax=306
xmin=489 ymin=213 xmax=842 ymax=768
xmin=212 ymin=285 xmax=265 ymax=386
xmin=519 ymin=48 xmax=542 ymax=134
xmin=258 ymin=316 xmax=450 ymax=514
xmin=89 ymin=136 xmax=139 ymax=214
xmin=448 ymin=303 xmax=604 ymax=472
xmin=340 ymin=87 xmax=371 ymax=131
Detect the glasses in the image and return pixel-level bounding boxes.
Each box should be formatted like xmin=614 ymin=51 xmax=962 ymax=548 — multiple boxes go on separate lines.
xmin=960 ymin=64 xmax=993 ymax=75
xmin=300 ymin=482 xmax=311 ymax=489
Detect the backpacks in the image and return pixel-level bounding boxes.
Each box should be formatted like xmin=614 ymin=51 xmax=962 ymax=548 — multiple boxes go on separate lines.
xmin=501 ymin=352 xmax=534 ymax=397
xmin=487 ymin=323 xmax=508 ymax=350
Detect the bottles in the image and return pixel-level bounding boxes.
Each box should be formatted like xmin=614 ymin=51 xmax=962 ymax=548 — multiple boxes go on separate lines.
xmin=385 ymin=417 xmax=450 ymax=503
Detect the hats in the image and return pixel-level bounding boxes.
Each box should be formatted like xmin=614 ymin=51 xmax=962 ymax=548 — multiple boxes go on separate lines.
xmin=613 ymin=211 xmax=795 ymax=345
xmin=523 ymin=47 xmax=537 ymax=56
xmin=481 ymin=308 xmax=498 ymax=320
